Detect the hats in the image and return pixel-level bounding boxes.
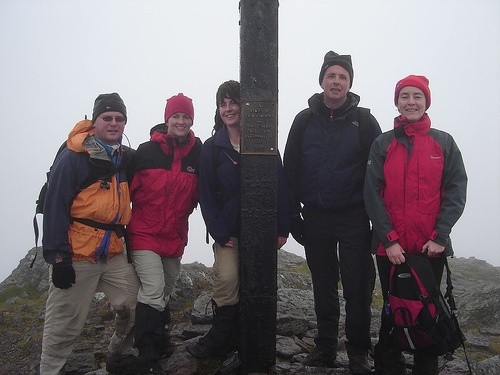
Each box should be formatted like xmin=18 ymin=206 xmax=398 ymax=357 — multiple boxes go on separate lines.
xmin=164 ymin=93 xmax=194 ymax=126
xmin=394 ymin=75 xmax=430 ymax=111
xmin=319 ymin=51 xmax=353 ymax=90
xmin=92 ymin=92 xmax=127 ymax=124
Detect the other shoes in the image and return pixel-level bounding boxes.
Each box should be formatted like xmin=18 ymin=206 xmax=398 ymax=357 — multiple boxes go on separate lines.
xmin=349 ymin=355 xmax=375 ymax=373
xmin=301 ymin=351 xmax=337 ymax=366
xmin=105 ymin=356 xmax=141 ymax=372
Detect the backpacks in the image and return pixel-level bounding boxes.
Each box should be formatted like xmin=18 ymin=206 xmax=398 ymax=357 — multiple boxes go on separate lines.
xmin=386 ymin=254 xmax=466 ymax=357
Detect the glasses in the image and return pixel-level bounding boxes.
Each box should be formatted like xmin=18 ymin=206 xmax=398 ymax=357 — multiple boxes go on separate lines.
xmin=98 ymin=115 xmax=126 ymax=122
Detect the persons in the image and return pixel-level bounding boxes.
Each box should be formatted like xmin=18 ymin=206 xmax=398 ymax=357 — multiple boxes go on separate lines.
xmin=127 ymin=92 xmax=203 ymax=375
xmin=38 ymin=92 xmax=142 ymax=375
xmin=281 ymin=51 xmax=385 ymax=375
xmin=186 ymin=80 xmax=291 ymax=362
xmin=363 ymin=75 xmax=467 ymax=375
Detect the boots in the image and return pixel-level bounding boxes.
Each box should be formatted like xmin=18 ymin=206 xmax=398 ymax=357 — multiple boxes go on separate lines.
xmin=134 ymin=300 xmax=166 ymax=375
xmin=186 ymin=298 xmax=240 ymax=357
xmin=159 ymin=304 xmax=174 ymax=358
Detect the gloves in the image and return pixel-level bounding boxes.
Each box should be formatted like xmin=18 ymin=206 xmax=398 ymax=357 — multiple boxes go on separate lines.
xmin=291 ymin=217 xmax=304 ymax=246
xmin=52 ymin=260 xmax=76 ymax=289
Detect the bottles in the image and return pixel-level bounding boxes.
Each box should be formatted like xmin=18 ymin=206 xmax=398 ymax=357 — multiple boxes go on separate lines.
xmin=385 ymin=297 xmax=391 ymax=317
